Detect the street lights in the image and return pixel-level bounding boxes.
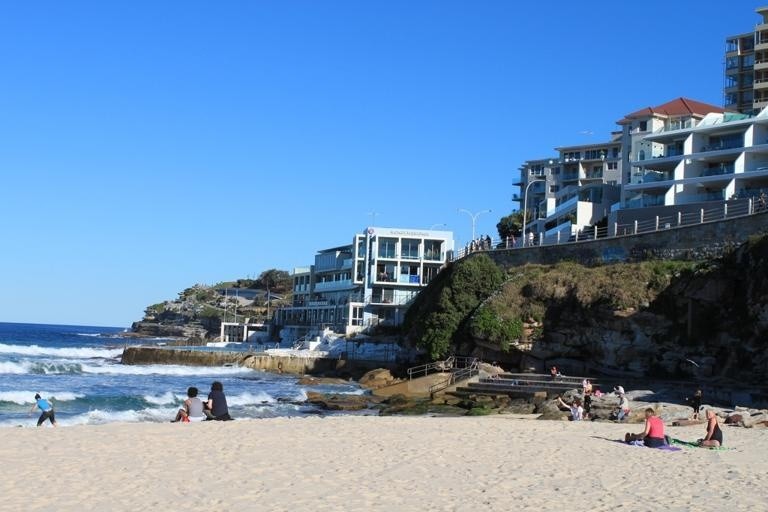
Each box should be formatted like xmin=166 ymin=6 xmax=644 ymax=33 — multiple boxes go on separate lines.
xmin=521 ymin=179 xmax=552 ymax=249
xmin=457 ymin=208 xmax=494 ymax=240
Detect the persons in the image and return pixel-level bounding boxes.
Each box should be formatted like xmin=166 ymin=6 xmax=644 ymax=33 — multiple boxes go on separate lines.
xmin=549 ymin=365 xmax=629 ymax=422
xmin=31 ymin=393 xmax=59 ymax=427
xmin=690 ymin=390 xmax=702 ymax=419
xmin=528 ymin=230 xmax=534 ymax=246
xmin=624 ymin=408 xmax=665 ymax=448
xmin=462 ymin=234 xmax=491 ymax=254
xmin=169 ymin=387 xmax=207 ymax=422
xmin=202 ymin=381 xmax=228 ymax=420
xmin=693 ymin=406 xmax=723 ymax=448
